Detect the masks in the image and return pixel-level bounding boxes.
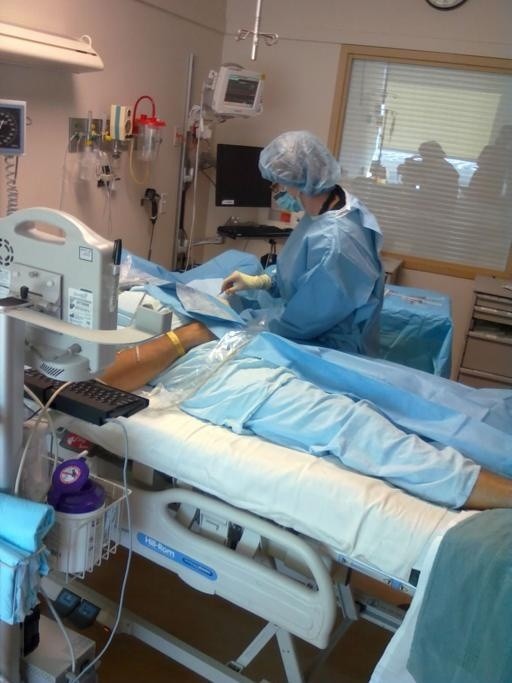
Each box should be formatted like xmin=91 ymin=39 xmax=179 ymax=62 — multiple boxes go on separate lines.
xmin=273 ymin=187 xmax=304 ymax=212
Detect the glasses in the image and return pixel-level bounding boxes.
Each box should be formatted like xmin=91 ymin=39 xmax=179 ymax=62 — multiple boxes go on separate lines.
xmin=270 ymin=182 xmax=279 ymax=193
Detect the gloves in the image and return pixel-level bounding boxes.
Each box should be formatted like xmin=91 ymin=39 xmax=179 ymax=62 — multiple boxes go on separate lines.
xmin=221 ymin=271 xmax=271 ymax=296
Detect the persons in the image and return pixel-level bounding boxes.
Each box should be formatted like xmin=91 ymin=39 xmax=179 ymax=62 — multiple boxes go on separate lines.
xmin=100 ymin=266 xmax=511 ymax=512
xmin=214 ymin=127 xmax=387 ymax=357
xmin=415 ymin=139 xmax=461 ymax=262
xmin=467 ymin=124 xmax=512 ymax=196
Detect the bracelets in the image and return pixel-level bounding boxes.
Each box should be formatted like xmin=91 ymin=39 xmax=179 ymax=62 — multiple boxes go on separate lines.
xmin=166 ymin=330 xmax=185 ymax=355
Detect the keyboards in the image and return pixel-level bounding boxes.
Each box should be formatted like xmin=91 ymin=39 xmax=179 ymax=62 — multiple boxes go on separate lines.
xmin=24 ymin=367 xmax=147 ymax=425
xmin=218 ymin=225 xmax=290 ymax=237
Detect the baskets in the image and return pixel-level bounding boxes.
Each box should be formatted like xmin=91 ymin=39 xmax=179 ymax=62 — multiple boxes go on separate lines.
xmin=9 ymin=452 xmax=132 ymax=586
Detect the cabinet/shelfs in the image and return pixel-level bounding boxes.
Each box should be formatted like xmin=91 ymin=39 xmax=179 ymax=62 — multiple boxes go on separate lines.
xmin=452 ymin=274 xmax=512 ymax=390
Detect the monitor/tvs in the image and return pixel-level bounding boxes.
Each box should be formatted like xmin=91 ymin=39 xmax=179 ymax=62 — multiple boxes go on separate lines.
xmin=215 ymin=143 xmax=272 ymax=208
xmin=211 ymin=66 xmax=264 ymax=116
xmin=0 ymin=100 xmax=26 ymax=154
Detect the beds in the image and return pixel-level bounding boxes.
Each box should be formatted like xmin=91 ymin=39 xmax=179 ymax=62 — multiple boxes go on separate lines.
xmin=24 ymin=370 xmax=512 ymax=682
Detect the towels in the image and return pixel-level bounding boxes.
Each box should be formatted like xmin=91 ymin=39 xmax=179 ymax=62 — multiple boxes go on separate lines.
xmin=407 ymin=506 xmax=512 ymax=682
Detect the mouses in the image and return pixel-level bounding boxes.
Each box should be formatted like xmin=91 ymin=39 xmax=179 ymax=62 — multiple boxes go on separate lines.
xmin=285 ymin=228 xmax=293 ymax=233
